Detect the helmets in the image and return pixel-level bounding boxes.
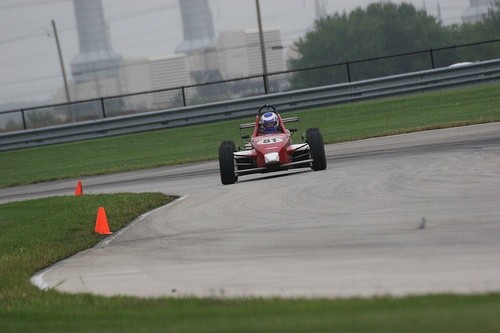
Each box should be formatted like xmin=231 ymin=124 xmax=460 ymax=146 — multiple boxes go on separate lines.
xmin=261 ymin=111 xmax=279 ymax=132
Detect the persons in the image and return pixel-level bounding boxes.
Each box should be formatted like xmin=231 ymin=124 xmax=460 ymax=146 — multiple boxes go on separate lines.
xmin=259 ymin=112 xmax=280 ymax=133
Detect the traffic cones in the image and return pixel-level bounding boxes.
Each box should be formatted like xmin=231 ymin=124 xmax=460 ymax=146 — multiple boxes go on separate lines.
xmin=94 ymin=207 xmax=114 ymax=235
xmin=74 ymin=181 xmax=84 ymax=196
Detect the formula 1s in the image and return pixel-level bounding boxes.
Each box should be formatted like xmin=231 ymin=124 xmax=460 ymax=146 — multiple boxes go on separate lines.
xmin=218 ymin=102 xmax=327 ymax=185
